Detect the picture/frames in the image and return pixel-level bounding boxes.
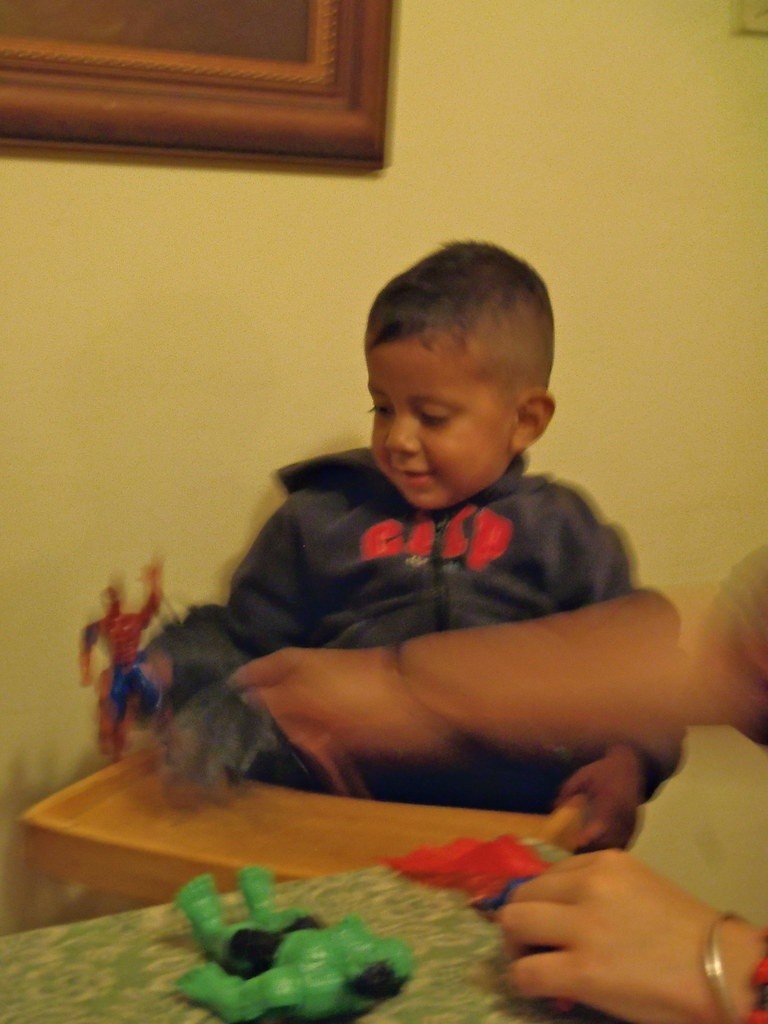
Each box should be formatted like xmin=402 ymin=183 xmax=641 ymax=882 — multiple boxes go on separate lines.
xmin=0 ymin=0 xmax=391 ymax=169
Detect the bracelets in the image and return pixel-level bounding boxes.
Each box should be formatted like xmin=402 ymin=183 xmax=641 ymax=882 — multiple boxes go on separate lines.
xmin=700 ymin=905 xmax=750 ymax=1022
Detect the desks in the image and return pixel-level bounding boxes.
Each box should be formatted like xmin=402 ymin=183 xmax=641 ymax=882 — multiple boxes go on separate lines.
xmin=1 ymin=840 xmax=597 ymax=1024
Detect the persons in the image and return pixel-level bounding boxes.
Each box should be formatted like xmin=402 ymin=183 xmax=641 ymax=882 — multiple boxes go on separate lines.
xmin=75 ymin=558 xmax=169 ymax=753
xmin=167 ymin=863 xmax=416 ymax=1024
xmin=219 ymin=547 xmax=768 ymax=1022
xmin=91 ymin=244 xmax=693 ymax=860
